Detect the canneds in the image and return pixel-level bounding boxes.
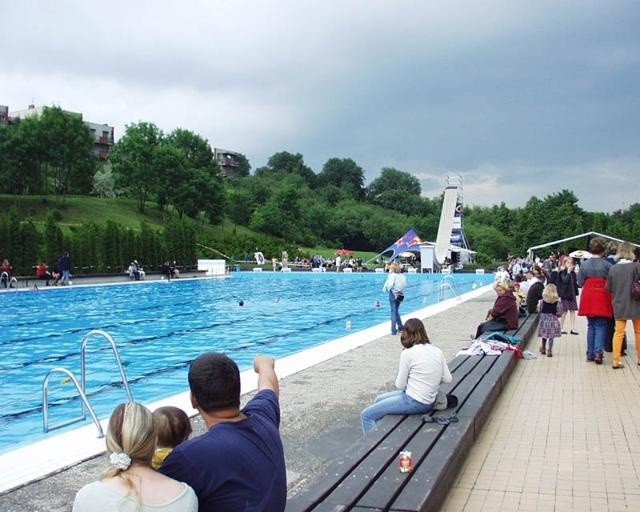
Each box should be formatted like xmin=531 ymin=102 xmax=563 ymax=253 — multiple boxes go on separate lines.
xmin=400 ymin=451 xmax=412 ymax=473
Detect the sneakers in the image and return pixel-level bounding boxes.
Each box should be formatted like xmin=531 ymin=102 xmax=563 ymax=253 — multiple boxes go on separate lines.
xmin=595 ymin=353 xmax=602 ymax=363
xmin=613 ymin=362 xmax=623 ymax=368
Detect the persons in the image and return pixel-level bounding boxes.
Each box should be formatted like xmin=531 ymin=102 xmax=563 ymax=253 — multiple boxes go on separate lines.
xmin=470 ymin=235 xmax=640 ymax=369
xmin=0 ymin=259 xmax=14 ymax=288
xmin=128 ymin=259 xmax=180 ymax=281
xmin=36 ymin=251 xmax=70 ymax=286
xmin=71 ymin=402 xmax=200 ymax=512
xmin=157 ymin=351 xmax=287 ymax=512
xmin=383 ymin=263 xmax=406 ymax=335
xmin=271 ymin=254 xmax=362 ymax=272
xmin=360 ymin=318 xmax=453 ymax=435
xmin=152 ymin=406 xmax=192 ymax=470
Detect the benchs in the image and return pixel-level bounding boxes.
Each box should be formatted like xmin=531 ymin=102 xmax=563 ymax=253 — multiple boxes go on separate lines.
xmin=283 ymin=414 xmax=475 ymax=511
xmin=429 ymin=346 xmax=522 ymax=439
xmin=1 ymin=268 xmax=209 ymax=288
xmin=494 ymin=304 xmax=542 ymax=346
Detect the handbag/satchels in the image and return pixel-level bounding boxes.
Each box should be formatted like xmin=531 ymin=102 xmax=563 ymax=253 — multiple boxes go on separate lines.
xmin=632 ymin=281 xmax=640 ymax=301
xmin=395 ymin=292 xmax=403 ymax=301
